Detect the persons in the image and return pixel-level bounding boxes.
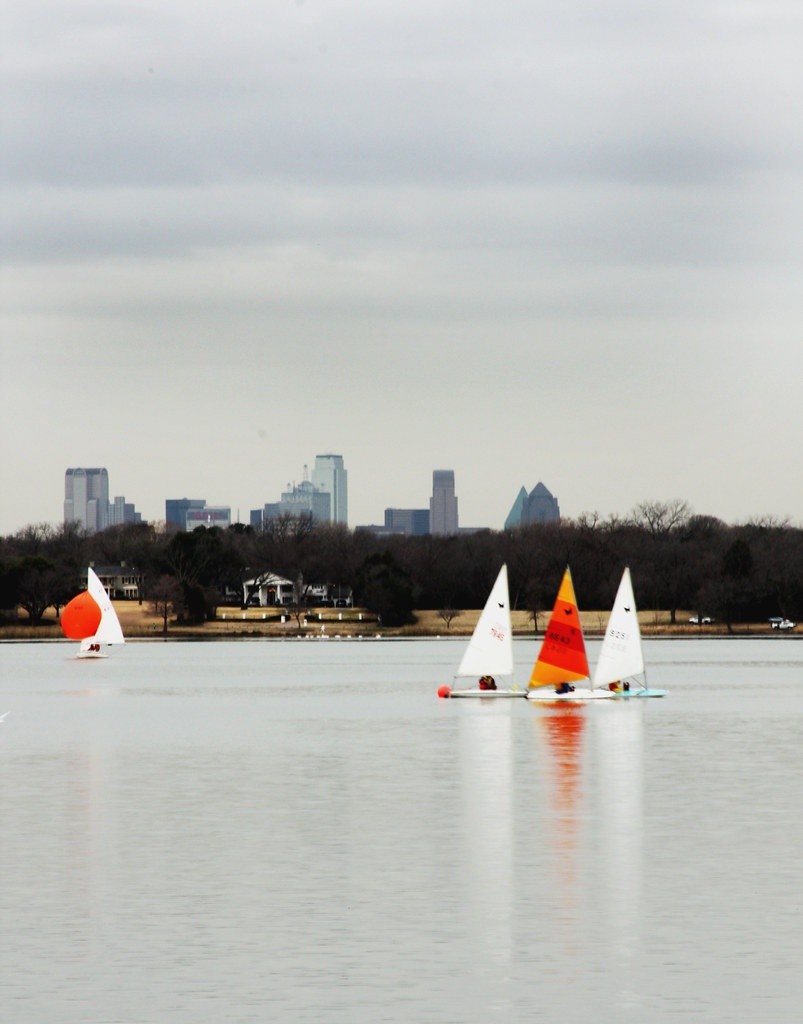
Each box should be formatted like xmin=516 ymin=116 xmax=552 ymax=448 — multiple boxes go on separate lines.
xmin=555 ymin=681 xmax=575 ymax=694
xmin=479 ymin=676 xmax=497 ymax=691
xmin=88 ymin=644 xmax=100 ymax=652
xmin=608 ymin=680 xmax=630 ymax=691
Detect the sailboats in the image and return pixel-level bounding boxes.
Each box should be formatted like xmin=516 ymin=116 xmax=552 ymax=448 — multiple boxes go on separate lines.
xmin=449 ymin=563 xmax=527 ymax=698
xmin=593 ymin=567 xmax=671 ymax=698
xmin=60 ymin=567 xmax=126 ymax=657
xmin=525 ymin=565 xmax=616 ymax=699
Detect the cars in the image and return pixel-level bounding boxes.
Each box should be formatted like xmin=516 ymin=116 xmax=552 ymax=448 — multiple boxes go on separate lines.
xmin=771 ymin=618 xmax=794 ymax=630
xmin=688 ymin=615 xmax=715 ymax=625
